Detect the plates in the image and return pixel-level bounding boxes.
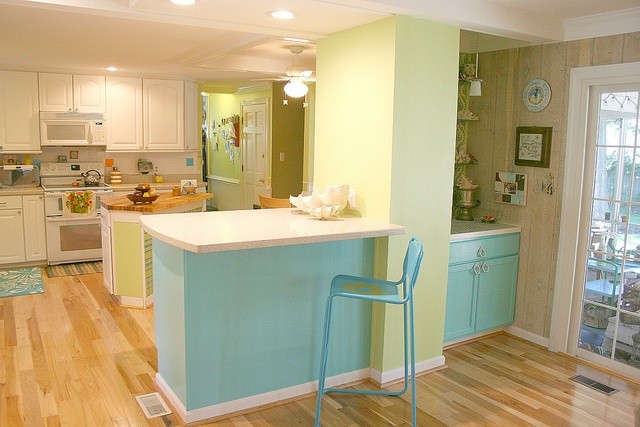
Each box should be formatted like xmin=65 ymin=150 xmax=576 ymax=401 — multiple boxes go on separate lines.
xmin=124 ymin=192 xmax=166 ymax=205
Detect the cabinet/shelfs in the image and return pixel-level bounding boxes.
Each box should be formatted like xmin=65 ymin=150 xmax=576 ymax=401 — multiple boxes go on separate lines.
xmin=22 ymin=195 xmax=48 ymax=262
xmin=0 ymin=209 xmax=26 ymax=265
xmin=185 ymin=81 xmax=198 ymax=153
xmin=0 ymin=69 xmax=42 ymax=157
xmin=39 ymin=71 xmax=103 ymax=114
xmin=440 ymin=229 xmax=519 ymax=345
xmin=105 ymin=76 xmax=185 ymax=152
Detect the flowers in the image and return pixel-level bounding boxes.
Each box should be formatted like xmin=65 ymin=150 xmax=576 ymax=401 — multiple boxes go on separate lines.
xmin=65 ymin=190 xmax=92 ymax=215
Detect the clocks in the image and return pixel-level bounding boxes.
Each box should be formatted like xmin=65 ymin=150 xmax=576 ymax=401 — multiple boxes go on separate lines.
xmin=523 ymin=78 xmax=552 ymax=112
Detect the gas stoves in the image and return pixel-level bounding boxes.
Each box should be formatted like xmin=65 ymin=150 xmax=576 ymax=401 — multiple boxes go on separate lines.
xmin=44 ymin=182 xmax=110 ymax=188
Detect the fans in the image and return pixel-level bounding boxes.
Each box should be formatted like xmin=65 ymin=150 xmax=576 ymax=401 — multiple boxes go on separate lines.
xmin=250 ymin=44 xmax=315 ymax=83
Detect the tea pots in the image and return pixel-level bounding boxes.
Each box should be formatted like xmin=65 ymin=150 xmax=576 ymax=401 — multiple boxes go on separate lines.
xmin=80 ymin=169 xmax=101 ymax=185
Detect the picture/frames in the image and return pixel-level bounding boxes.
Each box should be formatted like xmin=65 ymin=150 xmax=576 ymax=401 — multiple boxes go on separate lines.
xmin=515 ymin=124 xmax=552 ymax=169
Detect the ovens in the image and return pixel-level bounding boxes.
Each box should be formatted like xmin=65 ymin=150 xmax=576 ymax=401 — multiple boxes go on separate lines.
xmin=46 ymin=190 xmax=114 ymax=267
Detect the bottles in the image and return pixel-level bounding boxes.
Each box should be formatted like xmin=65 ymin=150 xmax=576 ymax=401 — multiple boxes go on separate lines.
xmin=110 ymin=166 xmax=121 ymax=185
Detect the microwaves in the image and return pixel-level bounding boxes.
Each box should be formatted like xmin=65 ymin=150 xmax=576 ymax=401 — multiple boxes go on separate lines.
xmin=40 ymin=111 xmax=108 ymax=147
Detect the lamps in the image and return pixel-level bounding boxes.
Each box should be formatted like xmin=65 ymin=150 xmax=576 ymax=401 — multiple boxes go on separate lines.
xmin=282 ymin=80 xmax=308 ymax=110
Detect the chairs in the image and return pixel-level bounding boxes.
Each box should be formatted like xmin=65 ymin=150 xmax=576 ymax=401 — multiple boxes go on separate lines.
xmin=585 ymin=256 xmax=622 ymax=317
xmin=316 ymin=236 xmax=423 ymax=427
